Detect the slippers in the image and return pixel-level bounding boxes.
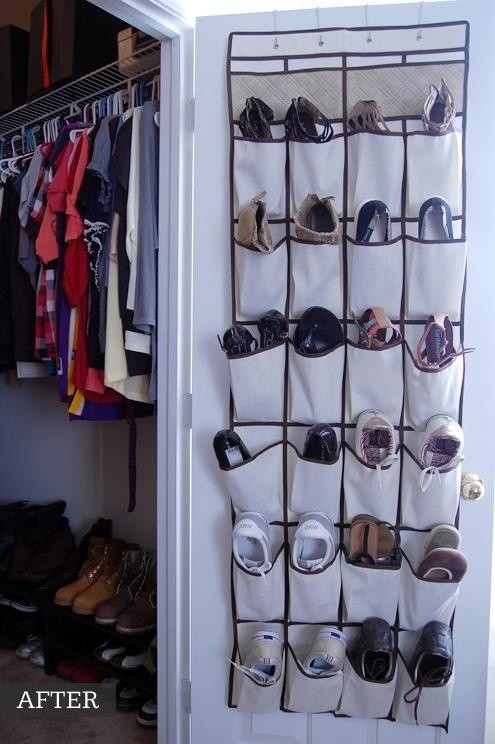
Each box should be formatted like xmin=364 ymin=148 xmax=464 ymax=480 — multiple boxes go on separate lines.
xmin=415 ymin=523 xmax=467 ymax=579
xmin=294 ymin=306 xmax=342 ymax=354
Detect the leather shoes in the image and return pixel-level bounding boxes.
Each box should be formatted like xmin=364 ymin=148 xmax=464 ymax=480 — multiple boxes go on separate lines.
xmin=302 ymin=423 xmax=337 ymax=461
xmin=213 ymin=429 xmax=252 ymax=469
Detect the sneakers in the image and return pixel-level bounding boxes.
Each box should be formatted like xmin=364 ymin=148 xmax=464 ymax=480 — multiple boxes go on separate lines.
xmin=351 ymin=618 xmax=396 ymax=683
xmin=412 ymin=621 xmax=453 ymax=686
xmin=294 ymin=192 xmax=339 ymax=243
xmin=291 ymin=512 xmax=336 ymax=572
xmin=353 ymin=199 xmax=392 ymax=242
xmin=236 ymin=191 xmax=272 ymax=253
xmin=0 ymin=499 xmax=158 ymax=728
xmin=355 ymin=410 xmax=395 ymax=467
xmin=231 ymin=513 xmax=271 ymax=575
xmin=418 ymin=414 xmax=464 ymax=472
xmin=418 ymin=196 xmax=453 ymax=240
xmin=244 ymin=632 xmax=283 ymax=683
xmin=302 ymin=628 xmax=348 ymax=677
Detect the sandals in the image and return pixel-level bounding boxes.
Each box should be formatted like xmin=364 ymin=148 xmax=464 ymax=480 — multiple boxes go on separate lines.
xmin=239 ymin=97 xmax=275 ymax=139
xmin=347 ymin=514 xmax=402 ymax=567
xmin=421 ymin=79 xmax=457 ymax=133
xmin=257 ymin=310 xmax=288 ymax=349
xmin=352 ymin=307 xmax=402 ymax=348
xmin=216 ymin=325 xmax=258 ymax=356
xmin=347 ymin=101 xmax=391 ymax=132
xmin=284 ymin=97 xmax=333 ymax=143
xmin=417 ymin=313 xmax=475 ymax=370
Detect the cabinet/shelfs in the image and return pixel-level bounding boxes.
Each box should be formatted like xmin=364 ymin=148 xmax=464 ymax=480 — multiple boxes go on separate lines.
xmin=0 ymin=567 xmax=157 ymax=698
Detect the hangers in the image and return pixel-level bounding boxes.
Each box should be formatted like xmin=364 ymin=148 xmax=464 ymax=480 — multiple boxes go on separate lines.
xmin=1 ymin=75 xmax=161 ymax=183
